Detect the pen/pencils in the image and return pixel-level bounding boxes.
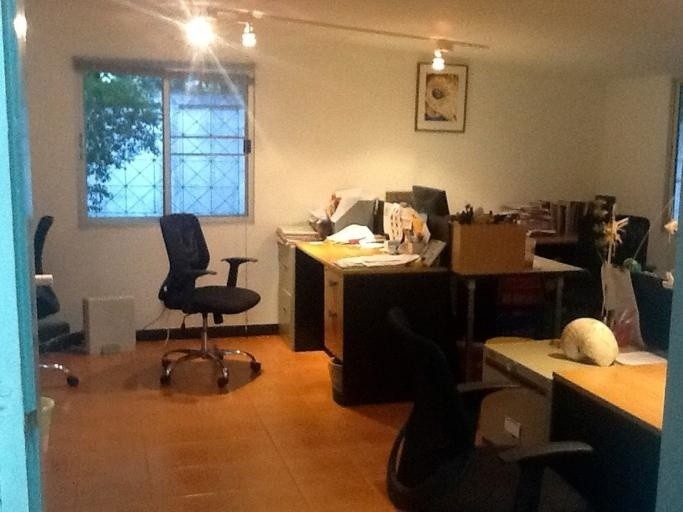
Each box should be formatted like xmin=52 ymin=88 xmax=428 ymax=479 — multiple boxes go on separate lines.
xmin=600 ymin=305 xmax=640 ymax=347
xmin=402 ymin=228 xmax=423 ymax=243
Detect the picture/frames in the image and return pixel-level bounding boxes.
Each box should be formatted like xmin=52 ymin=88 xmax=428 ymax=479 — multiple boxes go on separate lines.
xmin=416 ymin=59 xmax=469 ymax=134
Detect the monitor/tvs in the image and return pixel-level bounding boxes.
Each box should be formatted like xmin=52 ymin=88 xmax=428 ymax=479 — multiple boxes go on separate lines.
xmin=412 ymin=185 xmax=449 ymax=216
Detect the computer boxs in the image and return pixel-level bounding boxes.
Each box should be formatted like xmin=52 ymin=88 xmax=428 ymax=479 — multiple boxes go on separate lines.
xmin=82 ymin=296 xmax=137 ymax=354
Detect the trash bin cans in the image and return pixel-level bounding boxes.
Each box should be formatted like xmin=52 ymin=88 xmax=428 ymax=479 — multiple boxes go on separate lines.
xmin=39 ymin=396 xmax=55 ymax=453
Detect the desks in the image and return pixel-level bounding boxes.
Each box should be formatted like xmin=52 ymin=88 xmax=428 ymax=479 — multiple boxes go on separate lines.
xmin=277 ymin=208 xmax=463 ymax=404
xmin=472 ymin=332 xmax=673 ymax=508
xmin=454 ymin=216 xmax=590 ymax=392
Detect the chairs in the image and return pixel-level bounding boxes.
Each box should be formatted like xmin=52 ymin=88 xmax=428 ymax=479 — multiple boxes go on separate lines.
xmin=34 ymin=214 xmax=81 ymax=391
xmin=156 ymin=213 xmax=262 ymax=390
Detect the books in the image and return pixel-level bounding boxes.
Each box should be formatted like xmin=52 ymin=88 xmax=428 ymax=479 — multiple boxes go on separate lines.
xmin=276 ymin=223 xmax=322 ymax=244
xmin=504 ymin=191 xmax=617 ymax=239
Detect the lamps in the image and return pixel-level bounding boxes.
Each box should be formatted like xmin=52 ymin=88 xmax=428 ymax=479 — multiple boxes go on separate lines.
xmin=229 ymin=19 xmax=257 ymax=50
xmin=432 ymin=43 xmax=451 ymax=71
